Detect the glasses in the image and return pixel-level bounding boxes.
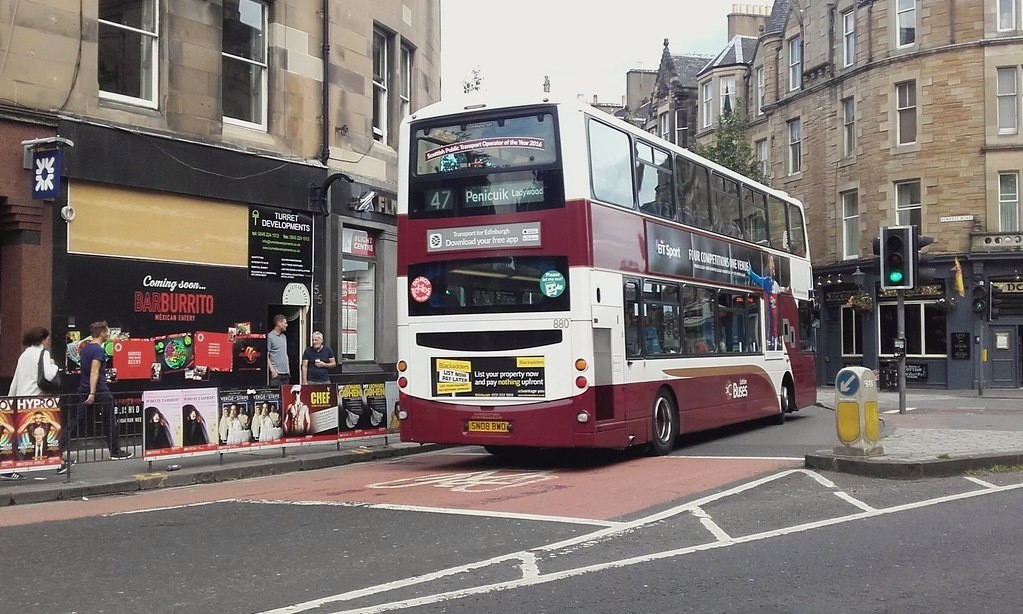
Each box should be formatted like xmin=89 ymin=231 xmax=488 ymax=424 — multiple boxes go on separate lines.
xmin=293 ymin=391 xmax=301 ymax=394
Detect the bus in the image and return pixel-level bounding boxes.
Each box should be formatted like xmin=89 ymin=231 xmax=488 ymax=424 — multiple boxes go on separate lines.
xmin=396 ymin=91 xmax=821 ymax=456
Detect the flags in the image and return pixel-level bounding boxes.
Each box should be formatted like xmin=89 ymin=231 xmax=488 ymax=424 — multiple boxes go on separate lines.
xmin=953 ymin=257 xmax=964 ymax=297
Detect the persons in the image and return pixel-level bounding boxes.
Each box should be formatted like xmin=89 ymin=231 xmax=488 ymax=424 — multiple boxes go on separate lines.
xmin=145 ymin=385 xmax=310 ymax=450
xmin=639 ymin=176 xmax=752 ymax=241
xmin=300 ymin=331 xmax=337 ymax=385
xmin=623 ymin=311 xmax=727 ymax=358
xmin=266 ymin=314 xmax=291 ymax=388
xmin=63 ymin=321 xmax=133 ymax=460
xmin=747 ymin=253 xmax=791 ymax=352
xmin=0 ymin=327 xmax=76 ymax=482
xmin=20 ymin=411 xmax=61 ymax=461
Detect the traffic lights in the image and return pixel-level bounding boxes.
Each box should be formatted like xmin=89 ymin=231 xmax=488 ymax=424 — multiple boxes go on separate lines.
xmin=990 ymin=282 xmax=1003 ymax=321
xmin=880 ymin=225 xmax=912 ymax=289
xmin=913 ymin=225 xmax=936 ymax=288
xmin=972 ymin=285 xmax=987 ymax=315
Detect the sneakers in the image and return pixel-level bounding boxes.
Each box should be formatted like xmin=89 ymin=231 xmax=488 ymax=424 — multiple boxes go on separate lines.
xmin=109 ymin=450 xmax=133 ymax=460
xmin=56 ymin=459 xmax=77 ymax=474
xmin=1 ymin=472 xmax=26 ymax=480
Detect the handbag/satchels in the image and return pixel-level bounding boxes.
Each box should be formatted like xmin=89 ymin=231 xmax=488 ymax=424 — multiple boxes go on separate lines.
xmin=38 ymin=350 xmax=62 ymax=394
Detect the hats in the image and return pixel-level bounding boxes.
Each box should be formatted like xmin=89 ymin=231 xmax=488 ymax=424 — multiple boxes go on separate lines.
xmin=290 ymin=385 xmax=301 ymax=394
